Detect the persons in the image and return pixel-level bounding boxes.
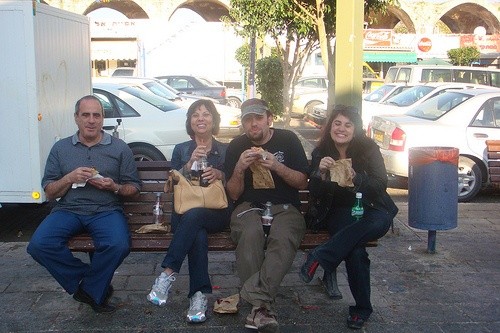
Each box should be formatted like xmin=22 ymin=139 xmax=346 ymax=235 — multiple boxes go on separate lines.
xmin=26 ymin=95 xmax=142 ymax=314
xmin=300 ymin=105 xmax=399 ymax=329
xmin=212 ymin=98 xmax=310 ymax=333
xmin=147 ymin=100 xmax=237 ymax=323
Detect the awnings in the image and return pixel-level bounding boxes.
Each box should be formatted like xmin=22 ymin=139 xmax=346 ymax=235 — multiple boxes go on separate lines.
xmin=479 ymin=53 xmax=500 ymax=64
xmin=92 ymin=40 xmax=138 ymax=61
xmin=364 ymin=51 xmax=417 ymax=63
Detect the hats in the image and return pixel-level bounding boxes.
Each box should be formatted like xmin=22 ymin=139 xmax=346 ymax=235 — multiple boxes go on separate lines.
xmin=240 ymin=104 xmax=269 ymax=120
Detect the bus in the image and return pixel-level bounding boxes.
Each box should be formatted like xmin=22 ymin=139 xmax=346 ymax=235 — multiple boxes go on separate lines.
xmin=384 ymin=65 xmax=500 ymax=88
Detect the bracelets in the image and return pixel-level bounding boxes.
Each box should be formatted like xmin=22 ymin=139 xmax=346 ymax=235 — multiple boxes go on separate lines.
xmin=114 ymin=184 xmax=120 ymax=194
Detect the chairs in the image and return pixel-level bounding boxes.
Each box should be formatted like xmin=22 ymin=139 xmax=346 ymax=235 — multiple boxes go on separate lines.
xmin=488 ymin=108 xmax=500 ymax=122
xmin=167 ymin=79 xmax=173 ymax=88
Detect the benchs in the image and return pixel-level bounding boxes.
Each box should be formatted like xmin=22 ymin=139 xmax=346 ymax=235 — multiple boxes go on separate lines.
xmin=64 ymin=161 xmax=379 ymax=305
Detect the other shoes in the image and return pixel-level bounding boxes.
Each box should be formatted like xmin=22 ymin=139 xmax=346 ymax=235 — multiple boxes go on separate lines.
xmin=73 ymin=289 xmax=114 ymax=314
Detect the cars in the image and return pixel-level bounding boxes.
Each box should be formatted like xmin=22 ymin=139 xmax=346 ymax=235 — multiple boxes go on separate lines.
xmin=287 ymin=76 xmax=329 ymax=116
xmin=111 ymin=67 xmax=135 ymax=77
xmin=93 ymin=78 xmax=242 ymax=118
xmin=156 ymin=76 xmax=228 ymax=106
xmin=308 ymin=84 xmax=417 ymax=124
xmin=93 ymin=82 xmax=243 ymax=161
xmin=362 ymin=83 xmax=499 ymax=136
xmin=199 ymin=78 xmax=247 ymax=108
xmin=367 ymin=88 xmax=500 ymax=202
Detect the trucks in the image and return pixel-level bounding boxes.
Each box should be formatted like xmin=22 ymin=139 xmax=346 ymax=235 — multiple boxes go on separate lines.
xmin=298 ymin=62 xmax=384 ymax=93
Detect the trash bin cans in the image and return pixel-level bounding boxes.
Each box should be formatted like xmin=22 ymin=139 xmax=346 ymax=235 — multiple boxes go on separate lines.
xmin=408 ymin=146 xmax=459 ymax=251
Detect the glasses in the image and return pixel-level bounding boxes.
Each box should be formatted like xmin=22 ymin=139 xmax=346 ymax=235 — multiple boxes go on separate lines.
xmin=334 ymin=105 xmax=359 ymax=115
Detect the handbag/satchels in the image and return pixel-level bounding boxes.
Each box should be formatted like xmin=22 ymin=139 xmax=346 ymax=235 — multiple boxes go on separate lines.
xmin=163 ymin=169 xmax=228 ymax=214
xmin=306 ymin=175 xmax=338 ymax=232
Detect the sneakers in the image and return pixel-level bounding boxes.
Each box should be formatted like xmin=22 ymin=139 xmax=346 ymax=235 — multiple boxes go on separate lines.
xmin=148 ymin=272 xmax=176 ymax=305
xmin=188 ymin=295 xmax=207 ymax=322
xmin=245 ymin=307 xmax=279 ymax=333
xmin=300 ymin=252 xmax=319 ymax=282
xmin=347 ymin=314 xmax=366 ymax=328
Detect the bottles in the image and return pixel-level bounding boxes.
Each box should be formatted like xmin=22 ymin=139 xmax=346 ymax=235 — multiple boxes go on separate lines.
xmin=350 ymin=193 xmax=364 ymax=222
xmin=262 ymin=202 xmax=274 ymax=237
xmin=152 ymin=193 xmax=163 ymax=224
xmin=198 ymin=148 xmax=210 ymax=186
